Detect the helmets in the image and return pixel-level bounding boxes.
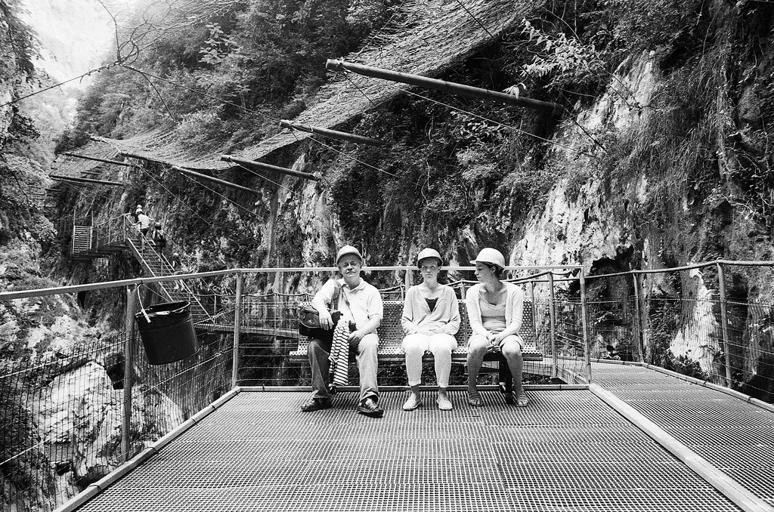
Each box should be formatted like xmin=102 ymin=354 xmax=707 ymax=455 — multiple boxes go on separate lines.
xmin=470 ymin=248 xmax=506 ymax=277
xmin=417 ymin=248 xmax=443 ymax=270
xmin=336 ymin=245 xmax=363 ymax=268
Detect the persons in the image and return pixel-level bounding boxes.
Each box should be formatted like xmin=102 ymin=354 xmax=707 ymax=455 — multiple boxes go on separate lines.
xmin=401 ymin=248 xmax=462 ymax=411
xmin=466 ymin=248 xmax=530 ymax=407
xmin=135 ymin=204 xmax=143 ymax=223
xmin=153 ymin=223 xmax=166 ymax=257
xmin=301 ymin=244 xmax=384 ymax=415
xmin=137 ymin=211 xmax=150 ymax=251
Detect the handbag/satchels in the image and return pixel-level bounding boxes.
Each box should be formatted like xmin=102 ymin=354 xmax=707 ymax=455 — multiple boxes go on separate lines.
xmin=298 ymin=305 xmax=343 ymax=343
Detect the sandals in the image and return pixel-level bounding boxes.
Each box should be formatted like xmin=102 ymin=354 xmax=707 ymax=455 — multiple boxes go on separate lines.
xmin=513 ymin=385 xmax=529 ymax=407
xmin=465 ymin=391 xmax=484 ymax=407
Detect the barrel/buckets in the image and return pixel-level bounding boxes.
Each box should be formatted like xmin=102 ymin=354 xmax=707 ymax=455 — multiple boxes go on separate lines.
xmin=134 ymin=283 xmax=199 ymax=365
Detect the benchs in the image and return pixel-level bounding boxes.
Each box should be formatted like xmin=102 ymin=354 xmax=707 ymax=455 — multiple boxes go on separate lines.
xmin=288 ymin=301 xmax=543 ymax=403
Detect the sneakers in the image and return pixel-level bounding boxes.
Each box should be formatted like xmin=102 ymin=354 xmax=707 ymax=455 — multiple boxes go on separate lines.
xmin=356 ymin=397 xmax=383 ymax=417
xmin=300 ymin=398 xmax=331 ymax=412
xmin=436 ymin=391 xmax=453 ymax=410
xmin=403 ymin=393 xmax=423 ymax=410
